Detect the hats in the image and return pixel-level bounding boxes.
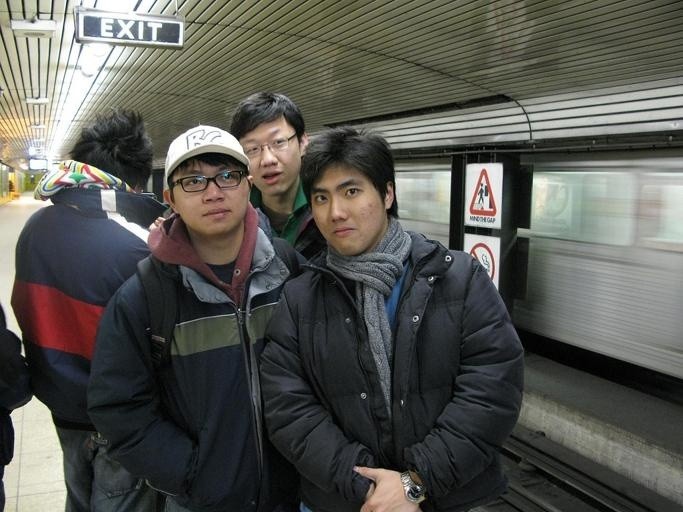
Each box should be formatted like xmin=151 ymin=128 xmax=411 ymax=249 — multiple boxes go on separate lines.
xmin=164 ymin=125 xmax=250 ymax=183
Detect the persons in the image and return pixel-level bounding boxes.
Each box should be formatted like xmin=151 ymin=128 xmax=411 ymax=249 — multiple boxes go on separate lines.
xmin=256 ymin=129 xmax=529 ymax=511
xmin=1 ymin=306 xmax=35 ymax=511
xmin=82 ymin=123 xmax=313 ymax=511
xmin=6 ymin=108 xmax=167 ymax=511
xmin=226 ymin=90 xmax=325 ymax=259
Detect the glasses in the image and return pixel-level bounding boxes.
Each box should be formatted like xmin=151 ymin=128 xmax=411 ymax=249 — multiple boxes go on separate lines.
xmin=242 ymin=131 xmax=295 ymax=157
xmin=170 ymin=170 xmax=247 ymax=192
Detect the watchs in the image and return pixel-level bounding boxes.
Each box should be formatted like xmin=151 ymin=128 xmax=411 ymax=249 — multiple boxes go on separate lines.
xmin=394 ymin=470 xmax=433 ymax=503
